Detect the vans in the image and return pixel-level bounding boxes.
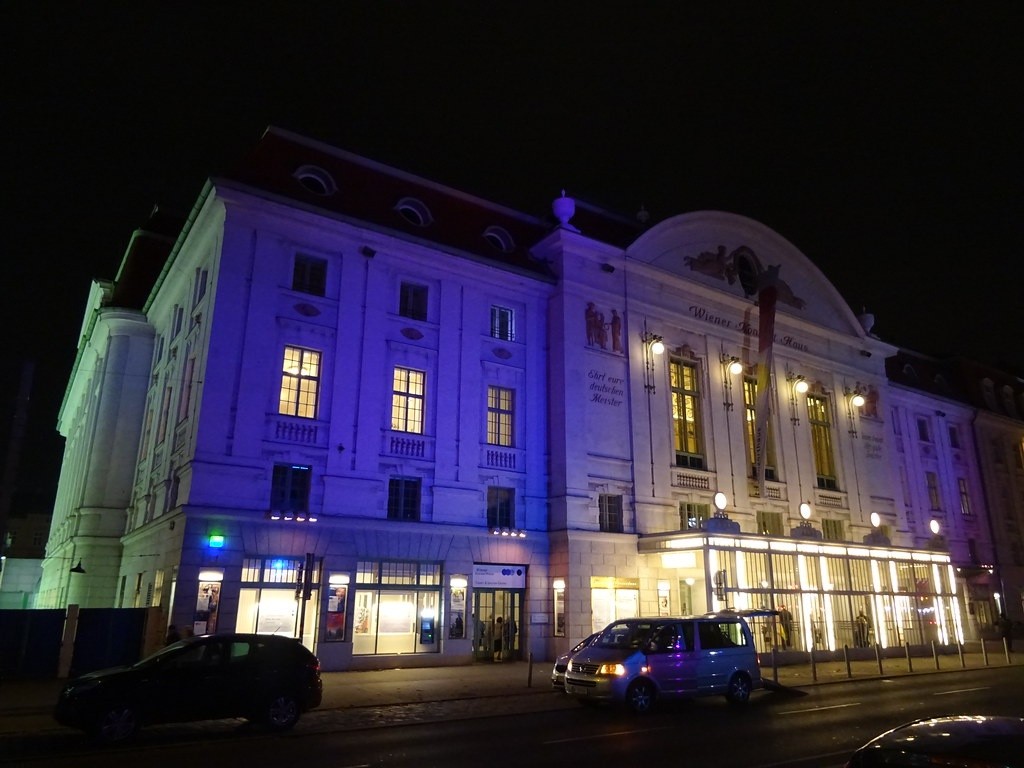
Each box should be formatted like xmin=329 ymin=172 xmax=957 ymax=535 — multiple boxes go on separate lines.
xmin=563 ymin=614 xmax=764 ymax=712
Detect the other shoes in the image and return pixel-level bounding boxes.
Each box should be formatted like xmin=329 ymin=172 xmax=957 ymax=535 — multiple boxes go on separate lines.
xmin=497 ymin=656 xmax=502 ymax=660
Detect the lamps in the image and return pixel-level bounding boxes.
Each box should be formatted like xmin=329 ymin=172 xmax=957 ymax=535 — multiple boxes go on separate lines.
xmin=640 ymin=317 xmax=665 ymax=395
xmin=718 ymin=339 xmax=743 ymax=411
xmin=799 ymin=502 xmax=812 ymax=519
xmin=712 ymin=490 xmax=728 ymax=510
xmin=845 ymin=381 xmax=868 ymax=441
xmin=786 ymin=366 xmax=810 ymax=428
xmin=492 ymin=527 xmax=527 ymax=538
xmin=268 ymin=511 xmax=318 ymax=522
xmin=870 ymin=511 xmax=881 ymax=527
xmin=930 ymin=519 xmax=940 ymax=534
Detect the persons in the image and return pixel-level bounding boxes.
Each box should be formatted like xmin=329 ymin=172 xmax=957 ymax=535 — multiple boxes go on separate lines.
xmin=472 ymin=612 xmax=517 ymax=660
xmin=779 ymin=605 xmax=794 ymax=646
xmin=166 ymin=624 xmax=202 ymax=662
xmin=998 ymin=613 xmax=1014 ymax=653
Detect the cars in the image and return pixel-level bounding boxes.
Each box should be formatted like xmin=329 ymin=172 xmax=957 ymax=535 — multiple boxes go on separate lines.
xmin=549 ymin=627 xmax=662 ymax=693
xmin=845 ymin=712 xmax=1024 ymax=768
xmin=53 ymin=631 xmax=324 ymax=743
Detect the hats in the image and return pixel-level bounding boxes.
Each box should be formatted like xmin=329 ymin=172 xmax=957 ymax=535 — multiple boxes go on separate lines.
xmin=780 ymin=605 xmax=785 ymax=607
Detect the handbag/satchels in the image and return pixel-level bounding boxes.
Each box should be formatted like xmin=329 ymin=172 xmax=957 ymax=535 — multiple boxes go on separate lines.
xmin=764 ymin=626 xmax=771 ymax=642
